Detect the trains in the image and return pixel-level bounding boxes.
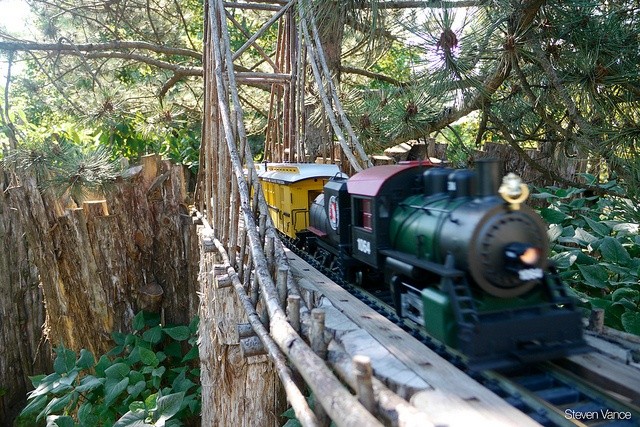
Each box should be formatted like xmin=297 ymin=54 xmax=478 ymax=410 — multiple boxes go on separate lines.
xmin=243 ymin=158 xmax=596 ymax=370
